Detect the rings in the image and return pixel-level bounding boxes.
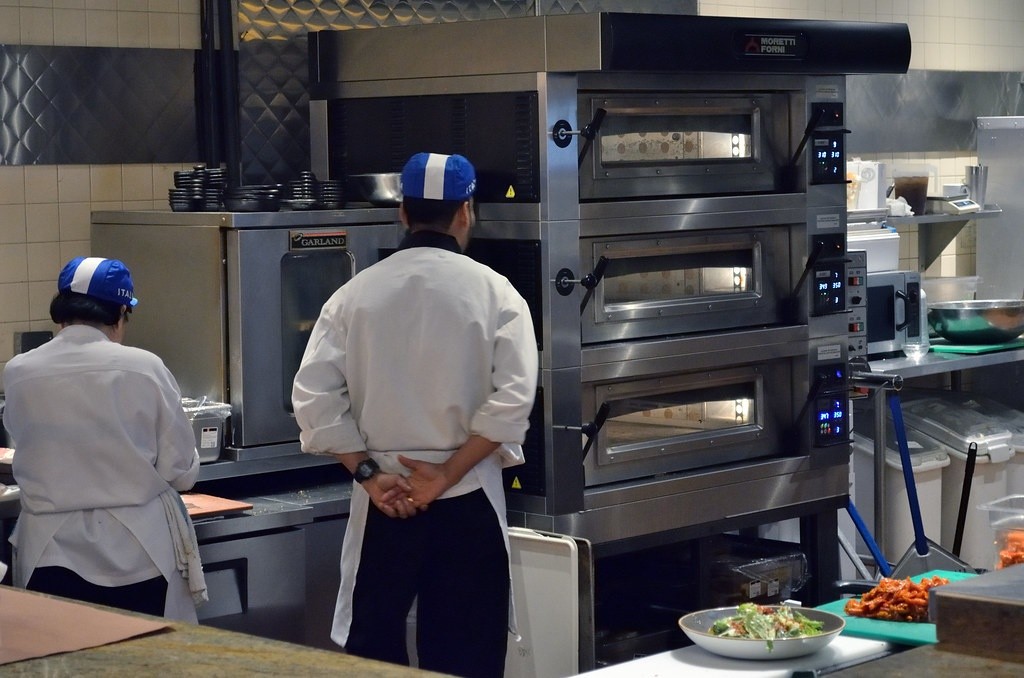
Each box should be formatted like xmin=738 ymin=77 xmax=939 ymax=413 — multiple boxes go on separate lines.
xmin=408 ymin=497 xmax=413 ymax=502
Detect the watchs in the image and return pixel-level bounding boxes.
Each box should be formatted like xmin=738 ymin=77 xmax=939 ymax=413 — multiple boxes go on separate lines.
xmin=352 ymin=459 xmax=380 ymax=484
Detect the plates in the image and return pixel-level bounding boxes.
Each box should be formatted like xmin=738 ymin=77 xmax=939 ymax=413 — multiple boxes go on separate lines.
xmin=168 ymin=168 xmax=342 ymax=212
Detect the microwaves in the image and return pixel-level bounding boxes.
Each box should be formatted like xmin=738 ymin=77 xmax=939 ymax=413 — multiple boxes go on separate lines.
xmin=866 ymin=271 xmax=928 ymax=354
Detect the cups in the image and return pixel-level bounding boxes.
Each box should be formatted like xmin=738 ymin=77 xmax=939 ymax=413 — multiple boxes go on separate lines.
xmin=965 ymin=165 xmax=988 ymax=210
xmin=892 ymin=162 xmax=930 ymax=215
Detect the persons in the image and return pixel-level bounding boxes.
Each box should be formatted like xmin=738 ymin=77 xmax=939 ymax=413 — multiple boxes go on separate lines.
xmin=2 ymin=257 xmax=209 ymax=626
xmin=291 ymin=152 xmax=539 ymax=678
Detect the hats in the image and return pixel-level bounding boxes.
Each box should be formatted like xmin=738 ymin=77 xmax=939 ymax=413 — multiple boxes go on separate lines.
xmin=400 ymin=152 xmax=476 ymax=200
xmin=58 ymin=256 xmax=139 ymax=306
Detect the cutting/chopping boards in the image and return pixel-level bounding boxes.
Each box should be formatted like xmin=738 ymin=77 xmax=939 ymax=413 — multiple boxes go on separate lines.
xmin=816 ymin=570 xmax=978 ymax=646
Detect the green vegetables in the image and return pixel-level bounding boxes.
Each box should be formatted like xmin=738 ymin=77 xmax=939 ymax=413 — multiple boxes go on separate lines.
xmin=711 ymin=603 xmax=826 ymax=639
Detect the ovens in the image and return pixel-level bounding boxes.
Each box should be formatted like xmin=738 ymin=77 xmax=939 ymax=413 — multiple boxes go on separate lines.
xmin=309 ymin=10 xmax=856 ymax=545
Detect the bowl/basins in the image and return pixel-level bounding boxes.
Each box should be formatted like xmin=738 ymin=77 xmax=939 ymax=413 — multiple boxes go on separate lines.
xmin=928 ymin=299 xmax=1024 ymax=344
xmin=901 ymin=342 xmax=930 ymax=358
xmin=976 ymin=494 xmax=1024 ymax=531
xmin=677 ymin=605 xmax=845 ymax=660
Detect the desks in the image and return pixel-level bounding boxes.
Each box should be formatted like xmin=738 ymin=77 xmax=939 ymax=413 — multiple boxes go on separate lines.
xmin=857 ymin=350 xmax=1024 ymax=583
